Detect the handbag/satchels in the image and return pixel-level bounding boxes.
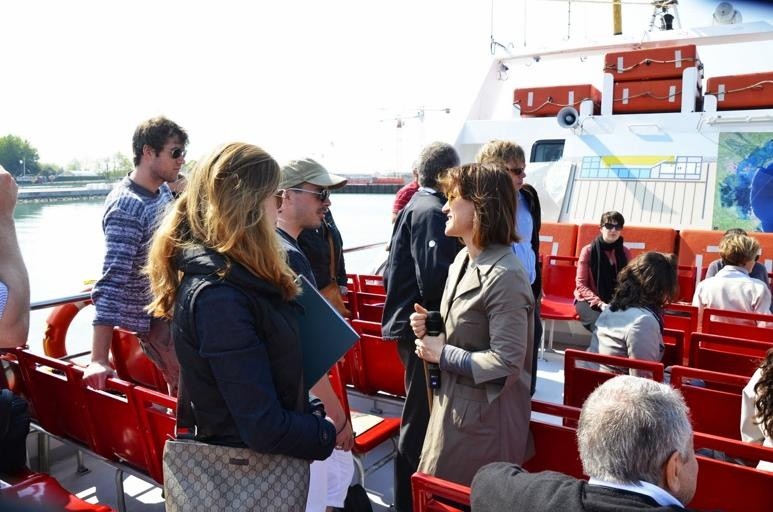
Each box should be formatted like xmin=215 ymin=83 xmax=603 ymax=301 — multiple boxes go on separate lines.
xmin=162 ymin=436 xmax=310 ymax=512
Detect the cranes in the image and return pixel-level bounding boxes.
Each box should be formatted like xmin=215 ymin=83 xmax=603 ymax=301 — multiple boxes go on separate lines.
xmin=363 ymin=97 xmax=450 ymax=132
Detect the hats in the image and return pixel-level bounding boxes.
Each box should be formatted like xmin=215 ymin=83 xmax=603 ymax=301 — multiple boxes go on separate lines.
xmin=276 ymin=156 xmax=348 ymax=194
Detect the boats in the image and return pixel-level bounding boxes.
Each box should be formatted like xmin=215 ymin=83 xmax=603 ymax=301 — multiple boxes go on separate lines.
xmin=0 ymin=0 xmax=773 ymax=512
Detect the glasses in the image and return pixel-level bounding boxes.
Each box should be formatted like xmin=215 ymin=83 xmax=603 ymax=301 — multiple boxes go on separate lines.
xmin=271 ymin=188 xmax=286 ymax=212
xmin=603 ymin=223 xmax=623 ymax=230
xmin=172 ymin=148 xmax=188 ymax=159
xmin=295 ymin=188 xmax=332 ymax=201
xmin=509 ymin=167 xmax=524 ymax=175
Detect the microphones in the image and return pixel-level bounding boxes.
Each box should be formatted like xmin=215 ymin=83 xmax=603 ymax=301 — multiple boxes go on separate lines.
xmin=425 ymin=311 xmax=442 ymax=388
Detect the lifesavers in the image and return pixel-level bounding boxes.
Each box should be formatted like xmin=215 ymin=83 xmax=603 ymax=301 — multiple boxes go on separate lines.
xmin=43 ymin=288 xmax=95 ymax=368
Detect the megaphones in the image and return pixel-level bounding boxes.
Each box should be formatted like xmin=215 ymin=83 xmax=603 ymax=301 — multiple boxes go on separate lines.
xmin=557 ymin=107 xmax=579 ymax=129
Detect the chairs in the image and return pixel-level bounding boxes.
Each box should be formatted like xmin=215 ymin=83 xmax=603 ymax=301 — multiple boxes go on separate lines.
xmin=408 ymin=398 xmax=772 ymax=510
xmin=560 ymin=304 xmax=773 ymax=428
xmin=1 ymin=269 xmax=405 ymax=511
xmin=540 ymin=251 xmax=772 ymax=356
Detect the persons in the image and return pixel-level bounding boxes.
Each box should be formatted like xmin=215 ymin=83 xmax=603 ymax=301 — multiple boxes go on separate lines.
xmin=571 ymin=249 xmax=707 ymax=389
xmin=475 ymin=139 xmax=543 ymax=399
xmin=703 ymin=227 xmax=769 ymax=289
xmin=81 ymin=114 xmax=192 ymax=418
xmin=381 ymin=140 xmax=466 ymax=512
xmin=466 ymin=370 xmax=702 ymax=512
xmin=570 ymin=209 xmax=634 ymax=337
xmin=687 ymin=233 xmax=771 ymax=331
xmin=735 ymin=347 xmax=773 ymax=474
xmin=0 ymin=160 xmax=34 ymax=355
xmin=409 ymin=160 xmax=536 ymax=487
xmin=139 ymin=142 xmax=374 ymax=510
